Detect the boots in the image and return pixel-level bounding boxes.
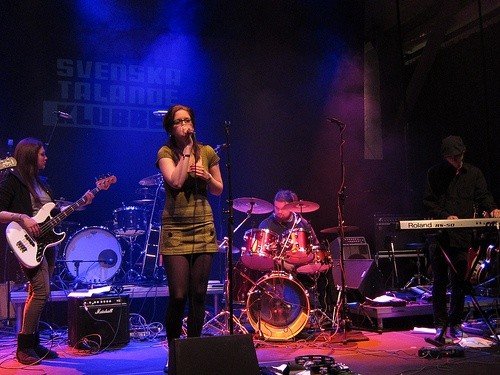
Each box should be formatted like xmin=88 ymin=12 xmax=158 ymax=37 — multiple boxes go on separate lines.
xmin=16 ymin=330 xmax=58 ymax=364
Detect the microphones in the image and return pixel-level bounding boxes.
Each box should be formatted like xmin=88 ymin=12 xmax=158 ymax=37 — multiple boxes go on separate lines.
xmin=214 ymin=143 xmax=230 ymax=152
xmin=328 ymin=117 xmax=345 ymax=125
xmin=282 ymin=303 xmax=291 ymax=311
xmin=54 ymin=111 xmax=72 ymax=118
xmin=153 ymin=110 xmax=168 ymax=116
xmin=105 ymin=257 xmax=115 ymax=265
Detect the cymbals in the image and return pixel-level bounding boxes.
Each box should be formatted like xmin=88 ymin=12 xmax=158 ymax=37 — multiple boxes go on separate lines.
xmin=128 ymin=198 xmax=160 ymax=205
xmin=282 ymin=200 xmax=322 ymax=213
xmin=138 ymin=172 xmax=166 ymax=187
xmin=54 ymin=198 xmax=87 ymax=211
xmin=230 ymin=197 xmax=276 ymax=215
xmin=319 ymin=224 xmax=363 ymax=235
xmin=214 ymin=238 xmax=240 ymax=254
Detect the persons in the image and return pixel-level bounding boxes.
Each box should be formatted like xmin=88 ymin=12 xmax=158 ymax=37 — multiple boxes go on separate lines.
xmin=0 ymin=136 xmax=95 ymax=365
xmin=417 ymin=136 xmax=500 ymax=339
xmin=259 ymin=190 xmax=320 ymax=288
xmin=155 ymin=105 xmax=223 ymax=371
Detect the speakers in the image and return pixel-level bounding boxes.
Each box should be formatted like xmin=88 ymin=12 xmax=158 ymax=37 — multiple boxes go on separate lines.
xmin=372 ymin=216 xmax=426 ymax=255
xmin=330 ymin=237 xmax=371 ymax=260
xmin=168 ymin=334 xmax=260 ymax=375
xmin=331 ymin=259 xmax=384 ymax=303
xmin=0 ymin=283 xmax=16 ymax=320
xmin=68 ymin=294 xmax=130 ymax=347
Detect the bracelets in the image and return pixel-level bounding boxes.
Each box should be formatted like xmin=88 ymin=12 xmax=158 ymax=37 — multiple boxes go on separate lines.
xmin=181 ymin=152 xmax=190 ymax=159
xmin=11 ymin=213 xmax=23 ymax=220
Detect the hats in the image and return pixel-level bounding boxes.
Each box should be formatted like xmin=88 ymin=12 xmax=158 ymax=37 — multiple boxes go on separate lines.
xmin=440 ymin=135 xmax=466 ymax=157
xmin=275 ymin=191 xmax=293 ymax=202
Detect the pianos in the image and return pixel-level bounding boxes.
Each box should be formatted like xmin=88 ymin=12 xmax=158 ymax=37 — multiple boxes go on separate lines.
xmin=391 ymin=217 xmax=500 ymax=348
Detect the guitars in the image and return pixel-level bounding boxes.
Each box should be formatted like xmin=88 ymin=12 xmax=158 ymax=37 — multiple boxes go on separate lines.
xmin=5 ymin=173 xmax=118 ymax=269
xmin=472 ymin=243 xmax=500 ymax=295
xmin=0 ymin=156 xmax=19 ymax=175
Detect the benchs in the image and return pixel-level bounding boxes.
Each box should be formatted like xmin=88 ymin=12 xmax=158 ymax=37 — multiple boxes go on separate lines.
xmin=10 ymin=283 xmax=222 ymax=333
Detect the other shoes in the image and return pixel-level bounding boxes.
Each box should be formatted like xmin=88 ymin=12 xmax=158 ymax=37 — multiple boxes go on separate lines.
xmin=433 ymin=328 xmax=446 ymax=339
xmin=164 ymin=365 xmax=174 ymax=373
xmin=451 ymin=327 xmax=467 ymax=338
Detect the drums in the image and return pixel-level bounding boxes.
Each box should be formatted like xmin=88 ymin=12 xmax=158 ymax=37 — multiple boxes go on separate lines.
xmin=297 ymin=244 xmax=333 ymax=274
xmin=62 ymin=225 xmax=126 ymax=287
xmin=244 ymin=271 xmax=312 ymax=342
xmin=240 ymin=227 xmax=279 ymax=272
xmin=281 ymin=226 xmax=316 ymax=266
xmin=110 ymin=206 xmax=151 ymax=239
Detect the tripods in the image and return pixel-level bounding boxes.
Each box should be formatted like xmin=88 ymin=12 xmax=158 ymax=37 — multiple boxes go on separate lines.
xmin=200 ymin=204 xmax=254 ymax=336
xmin=119 ymin=234 xmax=148 ymax=288
xmin=55 ymin=259 xmax=107 ymax=290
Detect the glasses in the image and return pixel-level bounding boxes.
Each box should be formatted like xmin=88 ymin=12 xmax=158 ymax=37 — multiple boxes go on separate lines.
xmin=173 ymin=118 xmax=192 ymax=125
